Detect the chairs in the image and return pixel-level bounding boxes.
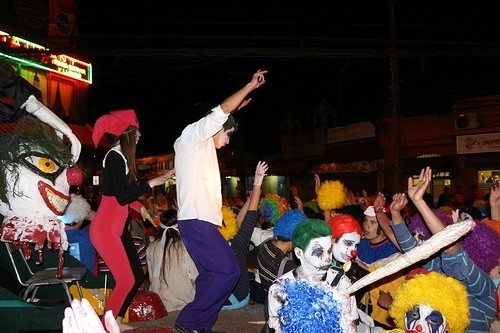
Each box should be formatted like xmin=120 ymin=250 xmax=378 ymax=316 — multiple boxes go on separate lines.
xmin=6 ymin=241 xmax=86 ymax=307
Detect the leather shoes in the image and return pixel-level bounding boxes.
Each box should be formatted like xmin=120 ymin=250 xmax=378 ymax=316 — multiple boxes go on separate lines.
xmin=174 ymin=323 xmax=214 ymax=333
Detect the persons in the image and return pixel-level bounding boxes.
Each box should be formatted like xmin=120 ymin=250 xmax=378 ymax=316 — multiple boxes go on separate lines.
xmin=87 ymin=110 xmax=176 ymax=333
xmin=62 ymin=298 xmax=119 ymax=333
xmin=389 ymin=166 xmax=496 ymax=332
xmin=276 ymin=278 xmax=344 ymax=333
xmin=82 ymin=174 xmax=500 ymax=286
xmin=360 ymin=272 xmax=410 ymax=333
xmin=268 ymin=218 xmax=359 ymax=333
xmin=174 ymin=68 xmax=269 ymax=333
xmin=220 ymin=158 xmax=268 ymax=312
xmin=145 ymin=209 xmax=199 ymax=313
xmin=386 ymin=273 xmax=471 ymax=332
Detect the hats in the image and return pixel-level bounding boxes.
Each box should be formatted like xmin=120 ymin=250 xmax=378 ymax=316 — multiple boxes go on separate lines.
xmin=92 ymin=109 xmax=139 ymax=149
xmin=273 ymin=208 xmax=308 ymax=239
xmin=364 ymin=205 xmax=385 ymax=216
